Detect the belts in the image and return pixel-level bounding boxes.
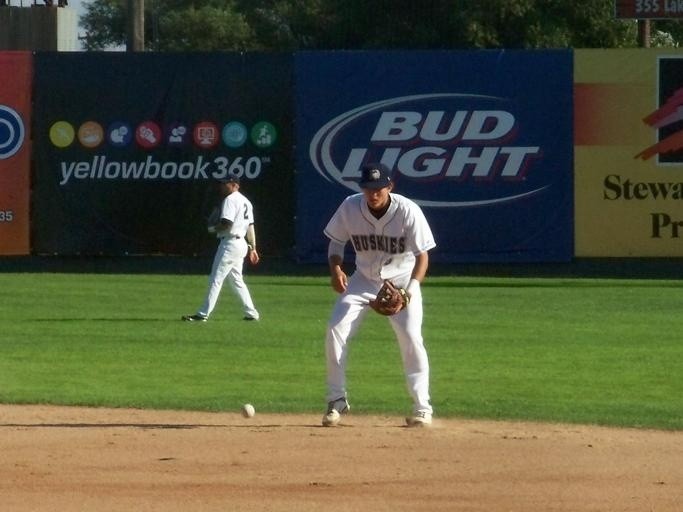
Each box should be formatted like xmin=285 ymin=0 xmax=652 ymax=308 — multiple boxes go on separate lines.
xmin=221 ymin=236 xmax=241 ymax=239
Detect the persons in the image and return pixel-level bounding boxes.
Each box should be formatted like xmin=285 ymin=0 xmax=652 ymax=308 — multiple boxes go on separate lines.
xmin=322 ymin=163 xmax=437 ymax=427
xmin=180 ymin=174 xmax=259 ymax=322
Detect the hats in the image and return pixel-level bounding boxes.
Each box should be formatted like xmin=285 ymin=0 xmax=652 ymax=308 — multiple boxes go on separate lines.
xmin=359 ymin=162 xmax=394 ymax=189
xmin=216 ymin=174 xmax=240 ymax=183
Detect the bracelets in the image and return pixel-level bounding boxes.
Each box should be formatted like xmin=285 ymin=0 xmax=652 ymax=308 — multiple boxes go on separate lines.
xmin=404 ymin=277 xmax=420 ymax=298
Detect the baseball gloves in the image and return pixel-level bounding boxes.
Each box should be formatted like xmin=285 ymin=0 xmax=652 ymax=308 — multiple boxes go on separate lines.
xmin=369 ymin=280 xmax=409 ymax=315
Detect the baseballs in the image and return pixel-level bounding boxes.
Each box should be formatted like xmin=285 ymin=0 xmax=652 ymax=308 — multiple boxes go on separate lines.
xmin=242 ymin=403 xmax=254 ymax=418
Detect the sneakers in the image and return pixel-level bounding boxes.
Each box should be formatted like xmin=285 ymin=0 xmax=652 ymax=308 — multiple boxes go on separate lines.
xmin=181 ymin=315 xmax=207 ymax=322
xmin=322 ymin=397 xmax=351 ymax=426
xmin=412 ymin=409 xmax=432 ymax=424
